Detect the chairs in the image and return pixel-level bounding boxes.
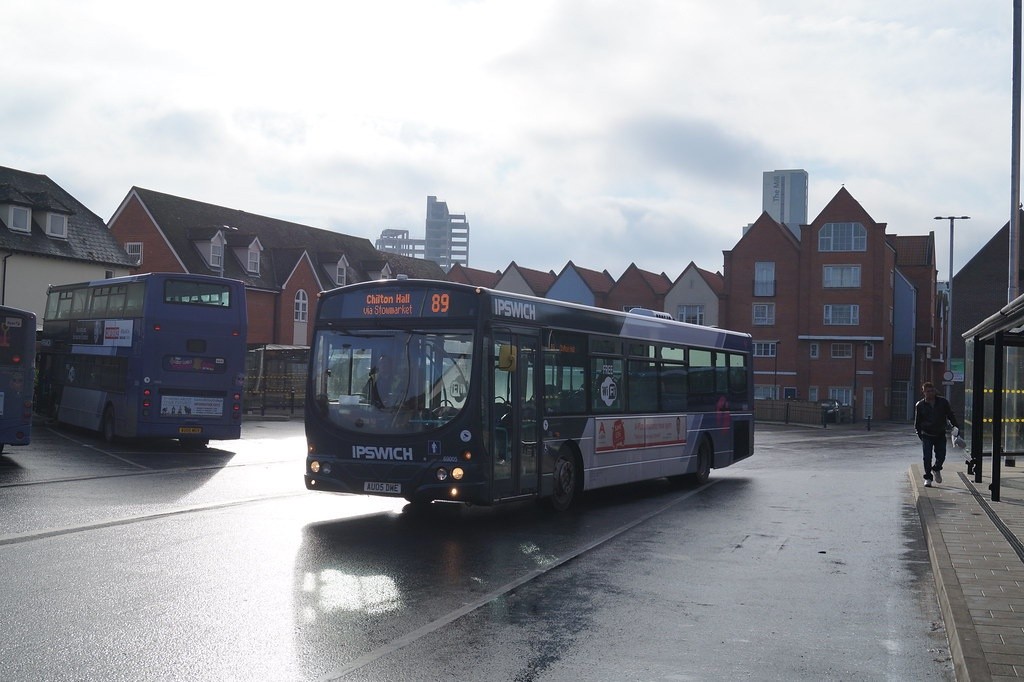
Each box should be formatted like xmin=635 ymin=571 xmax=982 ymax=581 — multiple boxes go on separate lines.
xmin=432 ymin=361 xmax=744 ymax=426
xmin=61 ymin=305 xmax=138 ymax=319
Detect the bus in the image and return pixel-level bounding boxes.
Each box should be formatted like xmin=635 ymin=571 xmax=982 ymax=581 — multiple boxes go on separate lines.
xmin=301 ymin=273 xmax=756 ymax=514
xmin=0 ymin=304 xmax=38 ymax=457
xmin=31 ymin=270 xmax=248 ymax=447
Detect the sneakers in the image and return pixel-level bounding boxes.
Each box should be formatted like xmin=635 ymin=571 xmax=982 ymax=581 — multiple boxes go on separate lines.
xmin=932 ymin=470 xmax=942 ymax=483
xmin=924 ymin=479 xmax=931 ymax=486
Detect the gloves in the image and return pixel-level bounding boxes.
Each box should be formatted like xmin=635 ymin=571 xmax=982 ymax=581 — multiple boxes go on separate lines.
xmin=918 ymin=433 xmax=923 ymax=441
xmin=955 ymin=429 xmax=960 ymax=437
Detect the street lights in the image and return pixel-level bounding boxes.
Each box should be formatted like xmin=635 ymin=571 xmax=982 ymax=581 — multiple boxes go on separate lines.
xmin=219 ymin=224 xmax=240 ymax=279
xmin=933 ymin=215 xmax=971 ymax=405
xmin=774 ymin=340 xmax=781 ymax=401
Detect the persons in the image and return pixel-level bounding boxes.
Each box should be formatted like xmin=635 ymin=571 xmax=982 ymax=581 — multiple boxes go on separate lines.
xmin=360 ymin=353 xmax=402 ymax=405
xmin=914 ymin=382 xmax=960 ymax=486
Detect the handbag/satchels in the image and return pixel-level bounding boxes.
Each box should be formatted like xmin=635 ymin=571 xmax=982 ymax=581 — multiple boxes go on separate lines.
xmin=951 ymin=435 xmax=966 ymax=448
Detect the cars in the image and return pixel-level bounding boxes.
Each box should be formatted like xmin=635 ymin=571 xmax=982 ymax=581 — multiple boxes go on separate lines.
xmin=817 ymin=399 xmax=842 ymax=422
